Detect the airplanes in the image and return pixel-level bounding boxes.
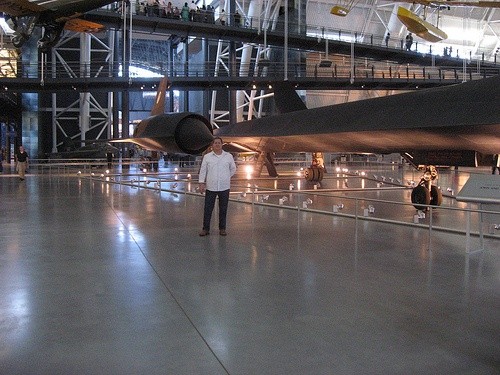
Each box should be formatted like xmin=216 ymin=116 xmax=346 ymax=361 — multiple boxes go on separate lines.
xmin=1 ymin=1 xmax=123 ymax=49
xmin=40 ymin=141 xmax=119 ymax=170
xmin=107 ymin=1 xmax=499 ymax=211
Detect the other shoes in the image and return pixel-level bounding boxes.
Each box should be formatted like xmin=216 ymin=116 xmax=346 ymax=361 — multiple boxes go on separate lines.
xmin=220 ymin=228 xmax=227 ymax=236
xmin=20 ymin=177 xmax=24 ymax=180
xmin=200 ymin=229 xmax=209 ymax=236
xmin=0 ymin=172 xmax=2 ymax=175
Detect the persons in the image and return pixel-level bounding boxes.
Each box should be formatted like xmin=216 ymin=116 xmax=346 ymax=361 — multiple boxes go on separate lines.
xmin=405 ymin=33 xmax=415 ymax=53
xmin=16 ymin=145 xmax=30 ymax=180
xmin=198 ymin=137 xmax=237 ymax=236
xmin=384 ymin=33 xmax=391 ymax=48
xmin=134 ymin=1 xmax=242 ymax=27
